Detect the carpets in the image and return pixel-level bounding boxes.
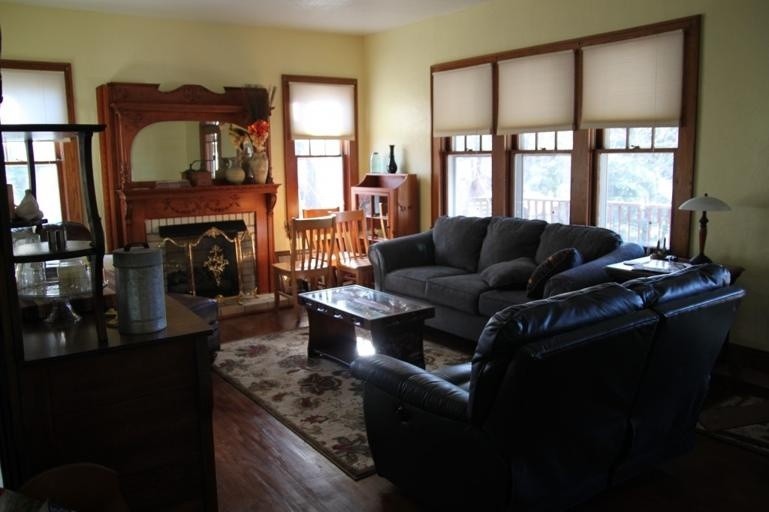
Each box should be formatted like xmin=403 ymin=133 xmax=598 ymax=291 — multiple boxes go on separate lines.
xmin=209 ymin=326 xmax=477 ymax=481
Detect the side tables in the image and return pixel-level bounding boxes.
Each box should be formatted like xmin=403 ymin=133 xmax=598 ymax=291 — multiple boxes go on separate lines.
xmin=607 ymin=250 xmax=699 ymax=287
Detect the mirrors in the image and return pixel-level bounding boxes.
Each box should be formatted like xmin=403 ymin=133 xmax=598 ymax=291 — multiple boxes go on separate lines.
xmin=117 ymin=119 xmax=242 ymax=183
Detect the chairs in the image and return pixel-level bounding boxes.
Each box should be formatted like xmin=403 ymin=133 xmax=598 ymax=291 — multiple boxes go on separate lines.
xmin=300 ymin=209 xmax=368 ymax=283
xmin=327 ymin=209 xmax=382 ymax=288
xmin=16 ymin=465 xmax=132 ymax=511
xmin=272 ymin=216 xmax=335 ymax=321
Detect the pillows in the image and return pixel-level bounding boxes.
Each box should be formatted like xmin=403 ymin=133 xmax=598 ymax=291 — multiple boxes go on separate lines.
xmin=534 ymin=223 xmax=616 ymax=264
xmin=481 ymin=257 xmax=537 ymax=289
xmin=434 ymin=217 xmax=490 ymax=272
xmin=478 ymin=217 xmax=544 ymax=275
xmin=525 ymin=246 xmax=582 ymax=297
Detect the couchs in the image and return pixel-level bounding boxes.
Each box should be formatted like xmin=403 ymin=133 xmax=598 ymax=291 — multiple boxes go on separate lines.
xmin=369 ymin=219 xmax=643 ymax=345
xmin=350 ymin=264 xmax=745 ymax=505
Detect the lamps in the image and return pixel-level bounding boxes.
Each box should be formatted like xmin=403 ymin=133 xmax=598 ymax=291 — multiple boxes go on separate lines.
xmin=678 ymin=193 xmax=731 ymax=265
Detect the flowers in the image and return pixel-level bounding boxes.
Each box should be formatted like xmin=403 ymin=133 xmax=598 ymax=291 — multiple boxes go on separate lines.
xmin=227 ymin=127 xmax=247 ymax=156
xmin=244 ymin=86 xmax=277 ymax=149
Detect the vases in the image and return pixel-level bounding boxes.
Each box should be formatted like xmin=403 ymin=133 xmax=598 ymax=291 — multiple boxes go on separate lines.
xmin=248 ymin=146 xmax=269 ymax=186
xmin=388 ymin=143 xmax=398 ymax=173
xmin=226 ymin=154 xmax=246 ymax=185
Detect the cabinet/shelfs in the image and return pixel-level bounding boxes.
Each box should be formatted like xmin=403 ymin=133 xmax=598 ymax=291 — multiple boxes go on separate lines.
xmin=5 ymin=295 xmax=220 ymax=507
xmin=1 ymin=124 xmax=110 ymax=347
xmin=349 ymin=174 xmax=418 ymax=257
